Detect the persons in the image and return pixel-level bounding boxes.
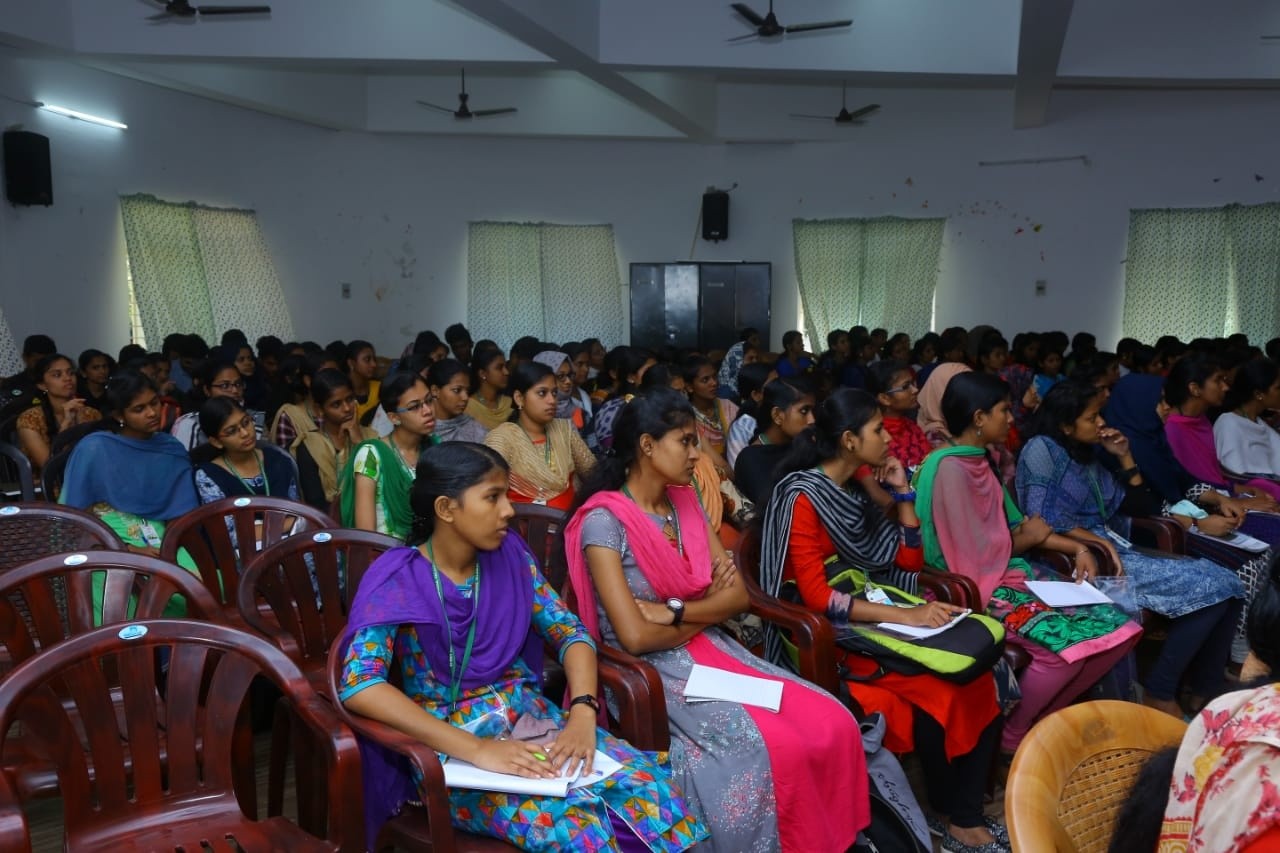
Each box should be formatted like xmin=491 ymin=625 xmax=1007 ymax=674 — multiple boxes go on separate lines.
xmin=0 ymin=322 xmax=1280 ymax=853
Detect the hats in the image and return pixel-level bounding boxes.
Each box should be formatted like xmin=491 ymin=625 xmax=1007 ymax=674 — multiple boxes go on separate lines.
xmin=180 ymin=333 xmax=209 ymax=355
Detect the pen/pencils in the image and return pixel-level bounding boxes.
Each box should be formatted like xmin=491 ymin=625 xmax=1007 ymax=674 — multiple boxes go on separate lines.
xmin=913 ymin=603 xmax=962 ymax=616
xmin=498 ymin=737 xmax=546 ymax=761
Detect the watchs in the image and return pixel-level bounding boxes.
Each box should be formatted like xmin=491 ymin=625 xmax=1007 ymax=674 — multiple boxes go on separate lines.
xmin=1188 ymin=518 xmax=1200 ymax=533
xmin=664 ymin=598 xmax=684 ymax=626
xmin=569 ymin=694 xmax=600 ymax=714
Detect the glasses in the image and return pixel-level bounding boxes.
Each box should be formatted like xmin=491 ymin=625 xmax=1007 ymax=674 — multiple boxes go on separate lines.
xmin=556 ymin=371 xmax=576 ymax=380
xmin=217 ymin=414 xmax=256 ymax=439
xmin=395 ymin=391 xmax=440 ymax=413
xmin=210 ymin=382 xmax=246 ymax=391
xmin=887 ymin=380 xmax=916 ymax=393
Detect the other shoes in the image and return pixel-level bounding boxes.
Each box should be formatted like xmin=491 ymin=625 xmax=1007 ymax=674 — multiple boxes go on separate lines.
xmin=941 ymin=826 xmax=1010 ymax=853
xmin=924 ymin=806 xmax=1011 ymax=846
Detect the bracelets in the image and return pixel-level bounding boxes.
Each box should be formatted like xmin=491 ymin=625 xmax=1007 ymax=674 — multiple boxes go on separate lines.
xmin=1076 ymin=547 xmax=1089 ymax=554
xmin=1124 ymin=463 xmax=1139 ymax=481
xmin=890 ymin=486 xmax=916 ymax=502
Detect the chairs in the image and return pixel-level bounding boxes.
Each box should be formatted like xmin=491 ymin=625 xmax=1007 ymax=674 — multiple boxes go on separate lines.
xmin=1 ymin=419 xmax=1188 ymax=853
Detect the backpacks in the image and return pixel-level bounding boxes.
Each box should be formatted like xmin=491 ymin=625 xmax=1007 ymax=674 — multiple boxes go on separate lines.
xmin=160 ymin=396 xmax=181 ymax=433
xmin=779 ymin=550 xmax=1006 ymax=682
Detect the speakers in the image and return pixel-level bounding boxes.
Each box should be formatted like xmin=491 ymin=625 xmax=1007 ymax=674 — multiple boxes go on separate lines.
xmin=702 ymin=192 xmax=729 ymax=239
xmin=4 ymin=131 xmax=54 ymax=206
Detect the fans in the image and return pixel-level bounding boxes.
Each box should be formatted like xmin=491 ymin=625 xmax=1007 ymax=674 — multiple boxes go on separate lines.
xmin=417 ymin=68 xmax=518 ymax=118
xmin=792 ymin=79 xmax=881 ymax=124
xmin=146 ymin=0 xmax=271 ymax=21
xmin=726 ymin=0 xmax=852 ymax=42
